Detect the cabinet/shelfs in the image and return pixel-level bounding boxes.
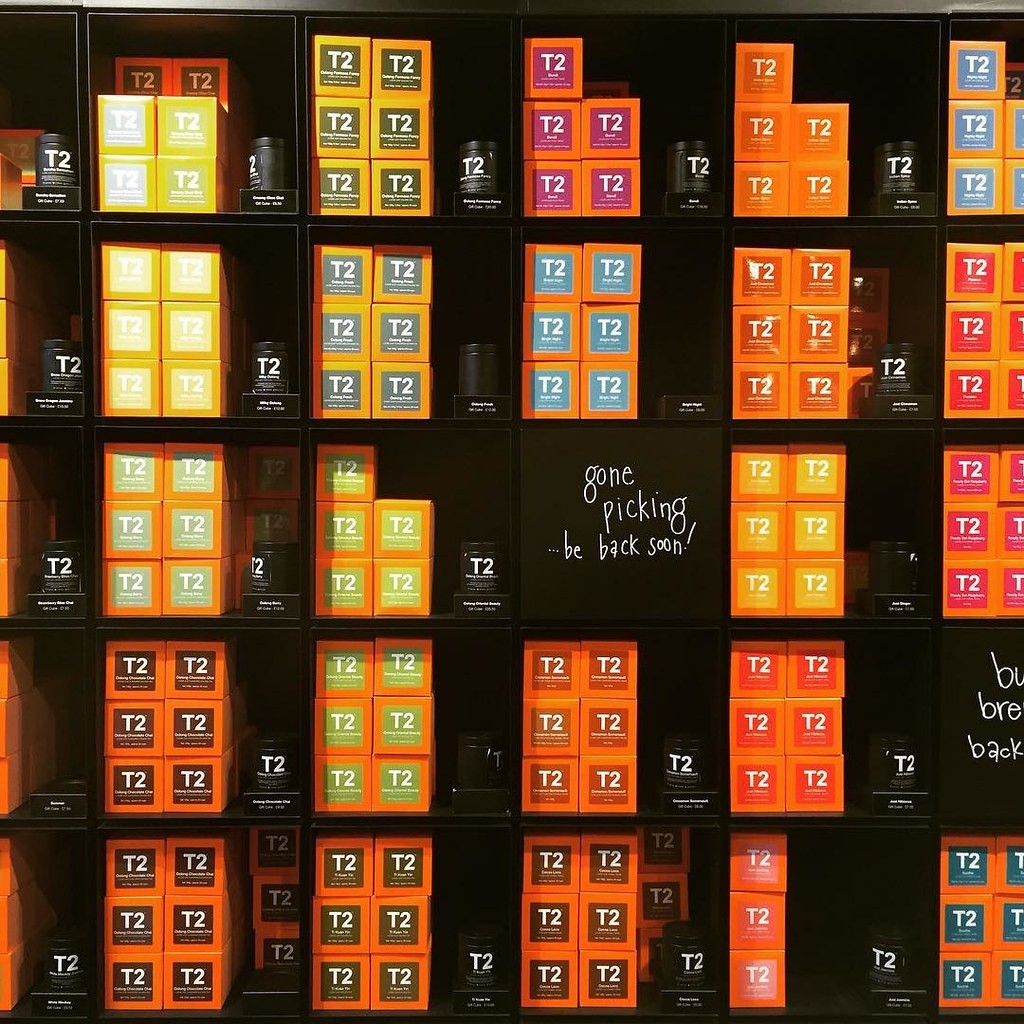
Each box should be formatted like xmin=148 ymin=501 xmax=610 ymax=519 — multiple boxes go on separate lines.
xmin=0 ymin=0 xmax=1024 ymax=1024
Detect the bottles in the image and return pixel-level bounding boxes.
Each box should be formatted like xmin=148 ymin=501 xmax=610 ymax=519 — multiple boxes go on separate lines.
xmin=460 ymin=140 xmax=501 ymax=193
xmin=457 ymin=730 xmax=504 ymax=789
xmin=251 ymin=341 xmax=289 ymax=394
xmin=253 ymin=732 xmax=297 ymax=791
xmin=870 ymin=540 xmax=919 ymax=595
xmin=39 ymin=541 xmax=82 ymax=593
xmin=663 ymin=920 xmax=712 ymax=989
xmin=459 ymin=343 xmax=500 ymax=394
xmin=662 ymin=734 xmax=705 ymax=789
xmin=35 ymin=133 xmax=77 ymax=187
xmin=252 ymin=541 xmax=298 ymax=594
xmin=888 ymin=737 xmax=918 ymax=791
xmin=670 ymin=140 xmax=715 ymax=191
xmin=39 ymin=338 xmax=83 ymax=391
xmin=460 ymin=920 xmax=507 ymax=987
xmin=46 ymin=929 xmax=88 ymax=990
xmin=248 ymin=136 xmax=292 ymax=189
xmin=865 ymin=917 xmax=918 ymax=987
xmin=874 ymin=141 xmax=919 ymax=193
xmin=876 ymin=343 xmax=917 ymax=394
xmin=460 ymin=541 xmax=504 ymax=593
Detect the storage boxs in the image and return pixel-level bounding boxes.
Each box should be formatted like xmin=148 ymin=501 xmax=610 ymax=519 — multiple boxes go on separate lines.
xmin=0 ymin=28 xmax=1024 ymax=1024
